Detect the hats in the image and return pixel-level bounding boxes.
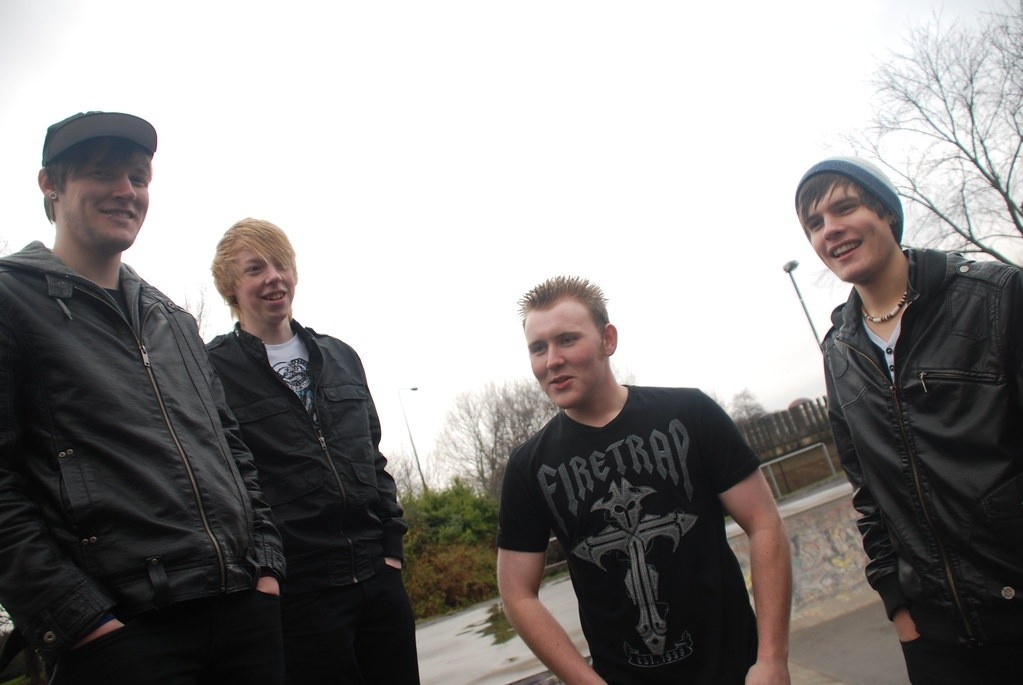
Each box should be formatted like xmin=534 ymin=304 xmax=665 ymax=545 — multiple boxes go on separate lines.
xmin=39 ymin=110 xmax=159 ymax=167
xmin=795 ymin=155 xmax=903 ymax=245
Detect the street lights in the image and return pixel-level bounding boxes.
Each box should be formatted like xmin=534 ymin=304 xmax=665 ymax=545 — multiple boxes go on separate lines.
xmin=398 ymin=386 xmax=439 ymax=496
xmin=783 ymin=259 xmax=823 ymax=351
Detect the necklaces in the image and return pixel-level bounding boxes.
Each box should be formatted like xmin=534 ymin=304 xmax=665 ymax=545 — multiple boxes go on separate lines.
xmin=860 ymin=291 xmax=908 ymax=324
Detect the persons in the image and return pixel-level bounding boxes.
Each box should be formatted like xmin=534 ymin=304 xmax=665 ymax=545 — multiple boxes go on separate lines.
xmin=1 ymin=111 xmax=288 ymax=684
xmin=794 ymin=156 xmax=1023 ymax=684
xmin=203 ymin=218 xmax=421 ymax=684
xmin=497 ymin=276 xmax=793 ymax=683
